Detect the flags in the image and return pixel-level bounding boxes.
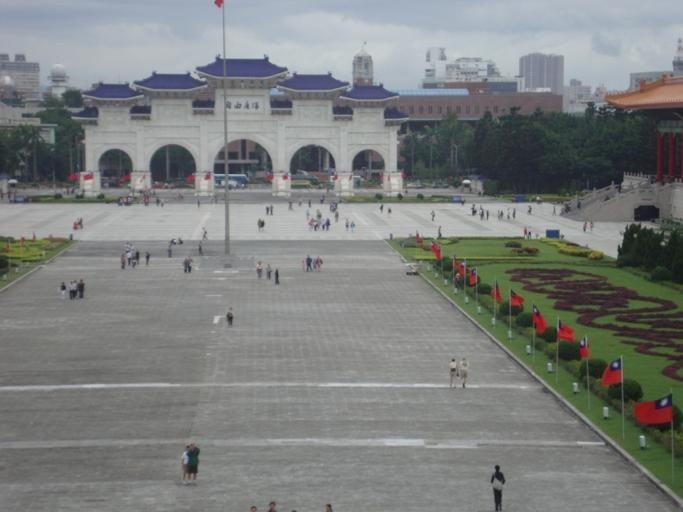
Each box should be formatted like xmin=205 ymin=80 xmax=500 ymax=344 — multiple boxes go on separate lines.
xmin=215 ymin=0 xmax=224 ymax=8
xmin=532 ymin=305 xmax=672 ymax=424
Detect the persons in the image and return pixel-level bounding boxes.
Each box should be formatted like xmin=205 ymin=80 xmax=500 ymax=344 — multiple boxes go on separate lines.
xmin=448 ymin=357 xmax=469 ymax=389
xmin=269 ymin=502 xmax=276 ymax=512
xmin=227 ymin=308 xmax=233 ymax=327
xmin=180 ymin=442 xmax=201 ymax=484
xmin=251 ymin=506 xmax=256 ymax=512
xmin=325 ymin=503 xmax=332 ymax=512
xmin=489 ymin=465 xmax=504 ymax=511
